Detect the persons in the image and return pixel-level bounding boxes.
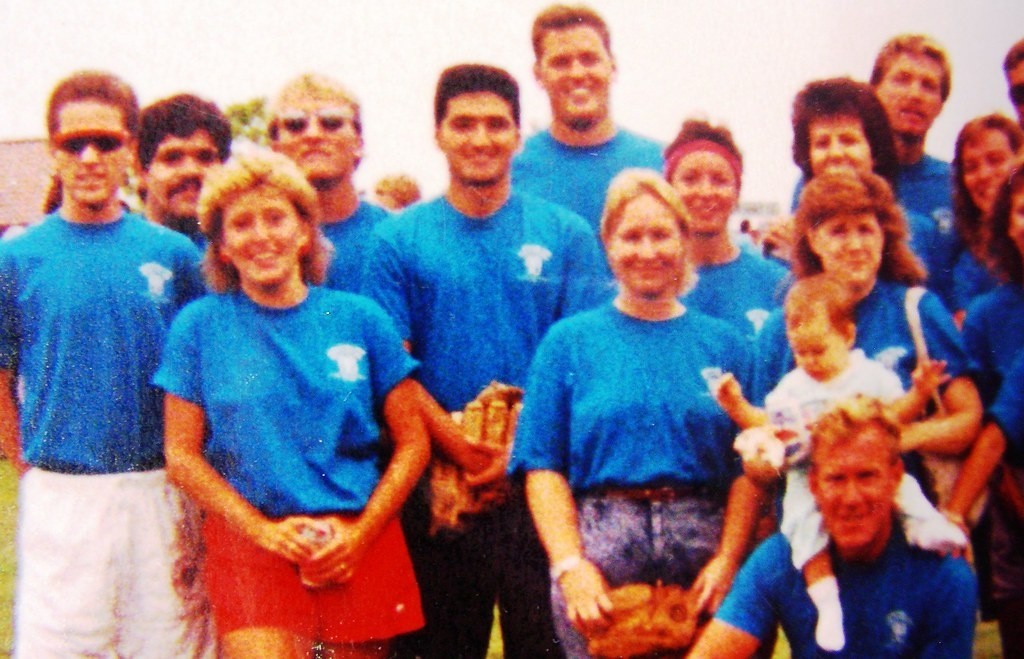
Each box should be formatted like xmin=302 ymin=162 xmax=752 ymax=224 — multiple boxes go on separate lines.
xmin=757 ymin=77 xmax=960 ymax=335
xmin=939 ymin=111 xmax=1023 ymax=333
xmin=502 ymin=167 xmax=769 ymax=659
xmin=660 ymin=117 xmax=792 ymax=348
xmin=735 ymin=165 xmax=984 ymax=570
xmin=263 ymin=72 xmax=392 ymax=294
xmin=787 ymin=33 xmax=966 ymax=266
xmin=510 ymin=3 xmax=666 ymax=281
xmin=707 ymin=272 xmax=968 ymax=652
xmin=371 ymin=173 xmax=421 ymax=213
xmin=358 ymin=63 xmax=610 ymax=658
xmin=0 ymin=69 xmax=216 ymax=659
xmin=1001 ymin=37 xmax=1024 ymax=129
xmin=137 ymin=93 xmax=232 ymax=257
xmin=146 ymin=145 xmax=431 ymax=658
xmin=935 ymin=345 xmax=1024 ymax=565
xmin=958 ymin=161 xmax=1024 ymax=401
xmin=684 ymin=391 xmax=980 ymax=659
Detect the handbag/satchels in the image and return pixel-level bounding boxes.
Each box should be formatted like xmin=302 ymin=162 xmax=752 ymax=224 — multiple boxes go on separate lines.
xmin=906 ymin=288 xmax=987 ymax=528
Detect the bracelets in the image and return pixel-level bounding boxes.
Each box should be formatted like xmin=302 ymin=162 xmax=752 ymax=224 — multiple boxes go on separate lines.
xmin=938 ymin=505 xmax=965 ymax=525
xmin=547 ymin=554 xmax=585 ymax=584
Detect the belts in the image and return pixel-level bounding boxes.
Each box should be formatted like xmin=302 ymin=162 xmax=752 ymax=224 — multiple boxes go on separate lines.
xmin=587 ymin=483 xmax=716 ymax=501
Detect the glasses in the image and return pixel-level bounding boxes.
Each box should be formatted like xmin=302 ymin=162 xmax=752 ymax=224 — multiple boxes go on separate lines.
xmin=55 ymin=131 xmax=129 ymax=154
xmin=279 ymin=111 xmax=352 ymax=134
